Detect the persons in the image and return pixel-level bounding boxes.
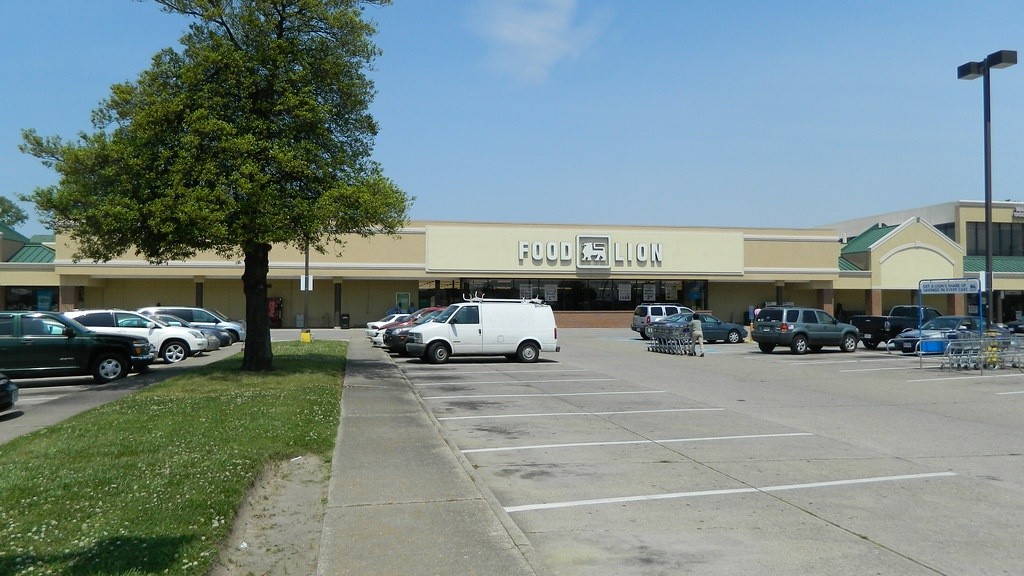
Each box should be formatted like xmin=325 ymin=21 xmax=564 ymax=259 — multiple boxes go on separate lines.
xmin=407 ymin=302 xmax=416 ymax=314
xmin=384 ymin=302 xmax=406 ymax=316
xmin=688 ymin=313 xmax=705 ymax=357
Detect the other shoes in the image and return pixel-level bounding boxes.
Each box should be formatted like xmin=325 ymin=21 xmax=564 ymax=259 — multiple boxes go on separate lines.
xmin=700 ymin=353 xmax=704 ymax=357
xmin=690 ymin=352 xmax=696 ymax=356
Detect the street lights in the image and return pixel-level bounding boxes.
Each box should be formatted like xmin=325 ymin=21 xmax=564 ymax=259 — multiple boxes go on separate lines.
xmin=958 ymin=49 xmax=1018 ymax=325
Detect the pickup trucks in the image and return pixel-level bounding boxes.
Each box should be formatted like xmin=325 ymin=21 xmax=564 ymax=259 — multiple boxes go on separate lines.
xmin=849 ymin=306 xmax=941 ymax=350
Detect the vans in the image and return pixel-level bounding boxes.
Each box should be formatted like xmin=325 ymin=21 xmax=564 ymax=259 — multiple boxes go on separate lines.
xmin=368 ymin=292 xmax=561 ymax=363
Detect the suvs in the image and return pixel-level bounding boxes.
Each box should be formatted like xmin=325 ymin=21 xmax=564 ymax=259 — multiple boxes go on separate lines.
xmin=632 ymin=303 xmax=695 ymax=340
xmin=0 ymin=306 xmax=248 ymax=412
xmin=750 ymin=306 xmax=860 ymax=355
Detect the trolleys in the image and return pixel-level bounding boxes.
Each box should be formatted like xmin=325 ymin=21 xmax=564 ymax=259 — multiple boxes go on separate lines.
xmin=645 ymin=323 xmax=696 ymax=356
xmin=940 ymin=330 xmax=1023 ymax=371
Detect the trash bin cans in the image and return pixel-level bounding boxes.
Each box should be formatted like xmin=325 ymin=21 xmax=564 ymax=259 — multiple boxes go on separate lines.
xmin=744 ymin=311 xmax=750 ymax=326
xmin=341 ymin=314 xmax=350 ymax=329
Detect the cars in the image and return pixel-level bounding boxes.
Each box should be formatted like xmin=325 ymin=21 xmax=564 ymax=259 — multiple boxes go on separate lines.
xmin=655 ymin=313 xmax=748 ymax=343
xmin=893 ymin=315 xmax=1024 ymax=351
